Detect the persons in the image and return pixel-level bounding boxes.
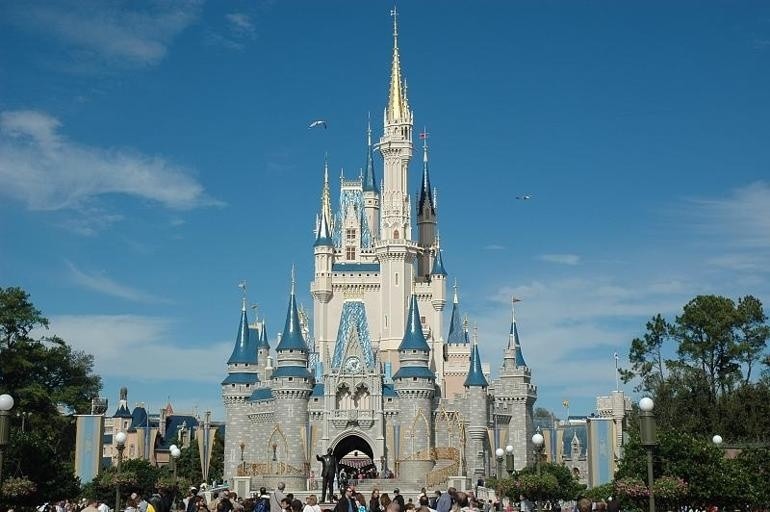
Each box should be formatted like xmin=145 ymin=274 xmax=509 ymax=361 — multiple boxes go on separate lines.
xmin=316 ymin=447 xmax=339 ymax=504
xmin=336 ymin=466 xmax=393 ymax=489
xmin=476 ymin=476 xmax=484 ymax=486
xmin=1 ymin=481 xmax=620 ymax=512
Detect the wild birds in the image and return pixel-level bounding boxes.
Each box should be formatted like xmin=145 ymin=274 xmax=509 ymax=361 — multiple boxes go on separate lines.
xmin=308 ymin=120 xmax=327 ymax=130
xmin=515 ymin=195 xmax=531 ymax=200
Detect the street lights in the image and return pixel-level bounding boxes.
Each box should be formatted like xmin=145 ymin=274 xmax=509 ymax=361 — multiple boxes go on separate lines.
xmin=532 ymin=425 xmax=544 ymax=512
xmin=169 ymin=444 xmax=181 ymax=480
xmin=17 ymin=412 xmax=33 ymax=432
xmin=496 ymin=445 xmax=514 ymax=512
xmin=115 ymin=432 xmax=127 ymax=512
xmin=639 ymin=397 xmax=657 ymax=512
xmin=0 ymin=394 xmax=15 ymax=490
